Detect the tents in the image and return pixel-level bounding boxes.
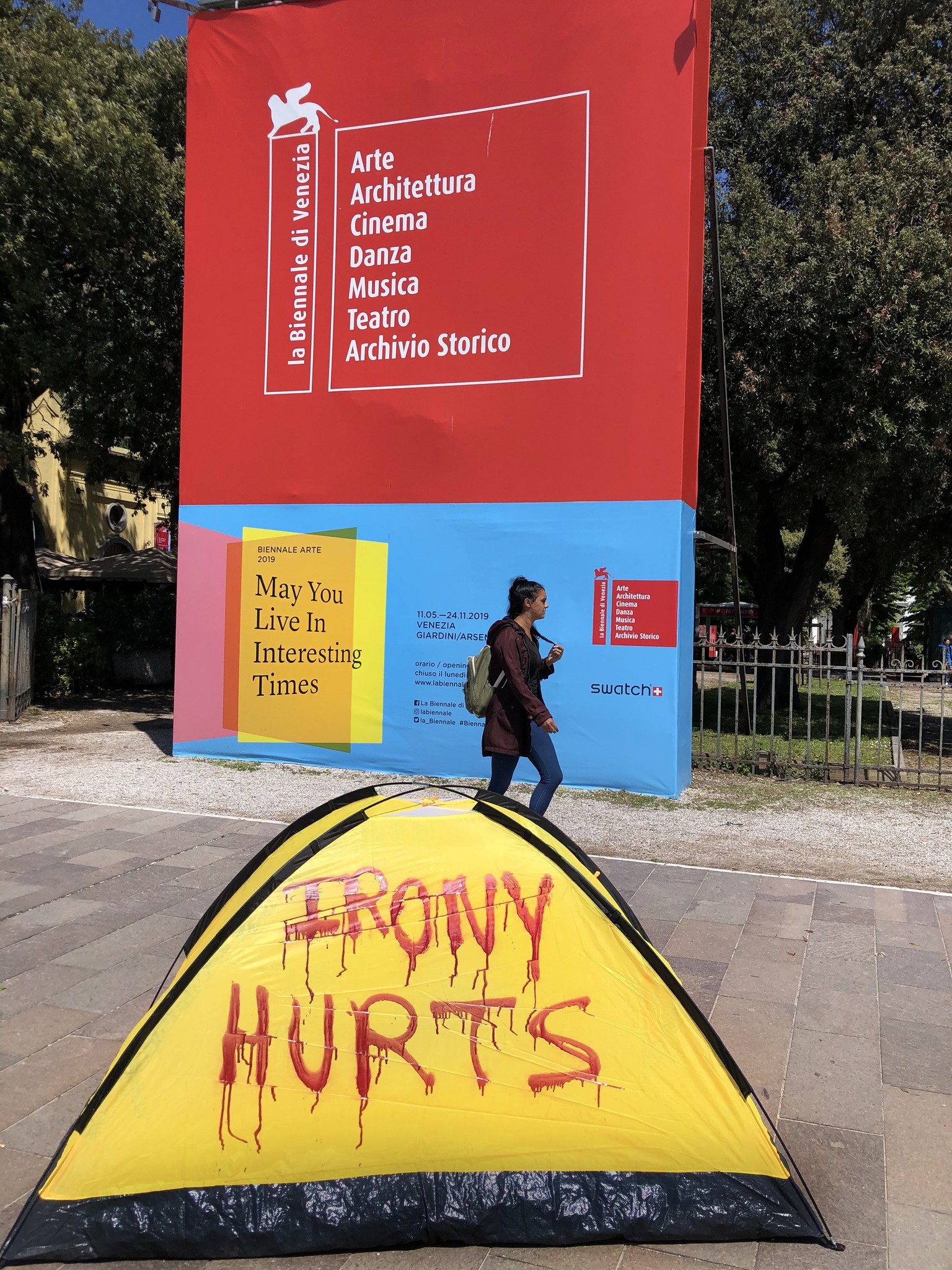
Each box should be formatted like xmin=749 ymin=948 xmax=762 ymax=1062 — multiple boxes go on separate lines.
xmin=3 ymin=780 xmax=832 ymax=1262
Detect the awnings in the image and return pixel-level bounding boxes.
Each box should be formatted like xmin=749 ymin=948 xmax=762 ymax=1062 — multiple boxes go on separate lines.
xmin=699 ymin=601 xmax=759 ymax=618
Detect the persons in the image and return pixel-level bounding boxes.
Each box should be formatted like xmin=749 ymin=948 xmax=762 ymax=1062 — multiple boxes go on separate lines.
xmin=481 ymin=577 xmax=564 ymax=819
xmin=938 ymin=635 xmax=952 ymax=685
xmin=712 ymin=628 xmax=754 ymax=668
xmin=906 ymin=640 xmax=914 ymax=670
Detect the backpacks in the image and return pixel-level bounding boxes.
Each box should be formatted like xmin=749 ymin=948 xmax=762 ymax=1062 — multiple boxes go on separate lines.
xmin=462 ymin=644 xmax=507 ymax=719
